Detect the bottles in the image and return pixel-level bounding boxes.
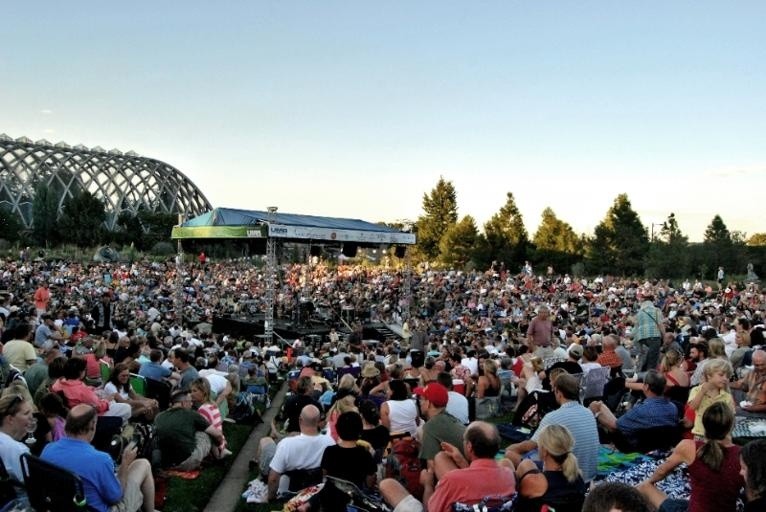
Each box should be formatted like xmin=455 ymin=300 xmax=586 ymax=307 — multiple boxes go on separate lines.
xmin=376 ymin=448 xmax=395 ymax=485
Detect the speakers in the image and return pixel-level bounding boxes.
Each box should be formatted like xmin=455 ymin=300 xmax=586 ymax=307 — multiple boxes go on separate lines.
xmin=223 ymin=313 xmax=231 ymax=318
xmin=341 ymin=242 xmax=357 ymax=257
xmin=259 ymin=319 xmax=265 ymax=324
xmin=310 ymin=246 xmax=321 ymax=257
xmin=394 ymin=246 xmax=406 ymax=258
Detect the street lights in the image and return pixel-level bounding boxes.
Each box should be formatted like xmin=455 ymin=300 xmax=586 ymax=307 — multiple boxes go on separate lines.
xmin=129 ymin=241 xmax=134 ymax=262
xmin=651 ymin=222 xmax=663 ymax=242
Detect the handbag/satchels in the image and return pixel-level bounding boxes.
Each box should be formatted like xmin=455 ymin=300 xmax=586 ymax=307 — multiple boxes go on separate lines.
xmin=387 ymin=438 xmax=424 ymax=496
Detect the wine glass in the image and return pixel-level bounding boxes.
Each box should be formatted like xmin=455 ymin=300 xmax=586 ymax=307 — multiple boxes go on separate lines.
xmin=24 ymin=419 xmax=38 ymax=443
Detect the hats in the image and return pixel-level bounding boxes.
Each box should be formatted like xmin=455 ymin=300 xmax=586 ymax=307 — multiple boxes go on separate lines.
xmin=568 ymin=343 xmax=583 ymax=357
xmin=361 ymin=364 xmax=380 ymax=378
xmin=413 ymin=384 xmax=448 ymax=405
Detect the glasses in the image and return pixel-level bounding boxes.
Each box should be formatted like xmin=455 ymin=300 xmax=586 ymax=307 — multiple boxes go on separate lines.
xmin=6 ymin=394 xmax=24 ymax=413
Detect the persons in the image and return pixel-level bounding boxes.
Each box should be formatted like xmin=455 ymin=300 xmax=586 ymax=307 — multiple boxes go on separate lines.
xmin=0 ymin=245 xmax=766 ymax=512
